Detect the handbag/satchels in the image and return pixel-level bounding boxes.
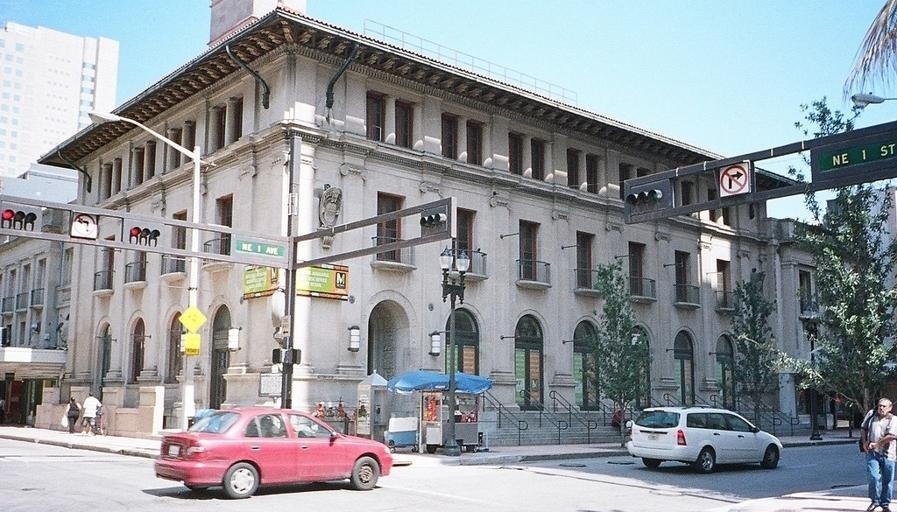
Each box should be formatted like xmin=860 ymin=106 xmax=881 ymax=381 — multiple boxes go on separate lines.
xmin=860 ymin=418 xmax=869 ymax=451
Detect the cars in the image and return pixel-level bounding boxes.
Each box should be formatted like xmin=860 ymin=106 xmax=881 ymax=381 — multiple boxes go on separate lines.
xmin=153 ymin=406 xmax=393 ymax=499
xmin=625 ymin=404 xmax=785 ymax=474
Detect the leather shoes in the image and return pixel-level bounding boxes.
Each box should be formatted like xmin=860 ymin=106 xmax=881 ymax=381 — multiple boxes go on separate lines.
xmin=867 ymin=504 xmax=891 ymax=512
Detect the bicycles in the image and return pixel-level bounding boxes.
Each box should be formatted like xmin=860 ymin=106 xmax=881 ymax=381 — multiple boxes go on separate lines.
xmin=91 ymin=411 xmax=106 ymax=435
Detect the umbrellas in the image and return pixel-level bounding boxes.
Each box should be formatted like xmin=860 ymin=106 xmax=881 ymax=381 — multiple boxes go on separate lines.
xmin=388 ymin=370 xmax=450 ymax=454
xmin=445 ymin=371 xmax=495 ymax=395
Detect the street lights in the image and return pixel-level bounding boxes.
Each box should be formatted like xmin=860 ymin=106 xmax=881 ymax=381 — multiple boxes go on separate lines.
xmin=804 ymin=320 xmax=824 ymax=440
xmin=87 ymin=108 xmax=215 ymax=439
xmin=438 ymin=243 xmax=472 ymax=456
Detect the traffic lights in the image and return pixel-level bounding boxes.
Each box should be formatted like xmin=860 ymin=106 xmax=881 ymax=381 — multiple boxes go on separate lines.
xmin=128 ymin=227 xmax=161 ymax=247
xmin=0 ymin=209 xmax=38 ymax=231
xmin=625 ymin=190 xmax=663 ymax=205
xmin=420 ymin=212 xmax=447 ymax=227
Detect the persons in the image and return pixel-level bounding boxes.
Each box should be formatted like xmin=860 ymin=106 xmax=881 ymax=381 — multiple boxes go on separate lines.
xmin=65 ymin=397 xmax=82 ymax=434
xmin=612 ymin=410 xmax=627 ymax=432
xmin=81 ymin=392 xmax=102 ymax=435
xmin=861 ymin=398 xmax=897 ymax=512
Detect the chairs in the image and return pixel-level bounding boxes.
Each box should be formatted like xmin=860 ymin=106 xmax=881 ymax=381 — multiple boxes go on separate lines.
xmin=691 ymin=414 xmax=724 ymax=428
xmin=249 ymin=416 xmax=280 ymax=438
